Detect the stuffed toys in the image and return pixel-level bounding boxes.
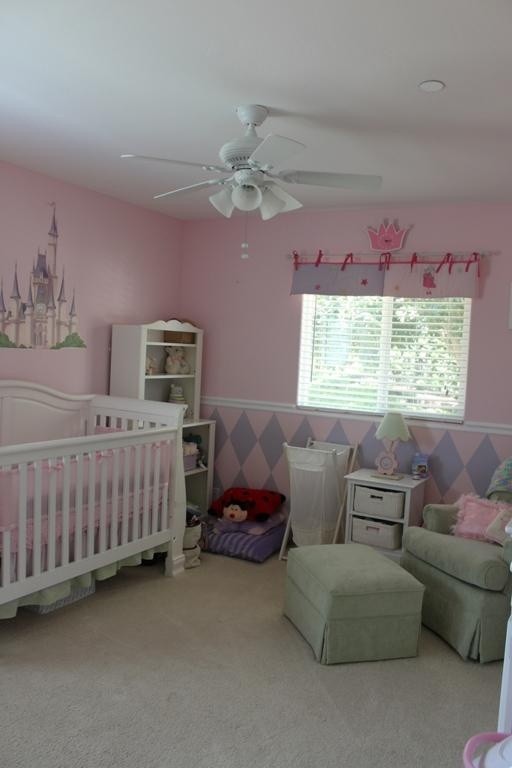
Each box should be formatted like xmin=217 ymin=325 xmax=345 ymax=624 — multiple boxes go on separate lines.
xmin=212 ymin=500 xmax=247 ymax=535
xmin=165 ymin=347 xmax=190 ymax=374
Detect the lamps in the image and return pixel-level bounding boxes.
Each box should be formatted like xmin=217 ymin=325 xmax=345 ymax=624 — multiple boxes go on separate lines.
xmin=208 ymin=172 xmax=284 ymax=264
xmin=370 ymin=413 xmax=413 ymax=482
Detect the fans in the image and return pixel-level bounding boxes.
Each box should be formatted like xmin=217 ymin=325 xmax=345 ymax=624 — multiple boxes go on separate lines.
xmin=120 ymin=103 xmax=384 ymax=225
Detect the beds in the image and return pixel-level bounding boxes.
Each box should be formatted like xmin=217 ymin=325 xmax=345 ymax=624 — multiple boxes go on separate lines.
xmin=0 ymin=380 xmax=189 ymax=620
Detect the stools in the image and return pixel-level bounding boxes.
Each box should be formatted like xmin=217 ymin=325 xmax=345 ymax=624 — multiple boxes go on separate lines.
xmin=278 ymin=541 xmax=426 ymax=666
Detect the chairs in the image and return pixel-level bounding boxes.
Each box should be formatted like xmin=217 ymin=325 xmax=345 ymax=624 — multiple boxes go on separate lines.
xmin=397 ymin=458 xmax=512 ymax=667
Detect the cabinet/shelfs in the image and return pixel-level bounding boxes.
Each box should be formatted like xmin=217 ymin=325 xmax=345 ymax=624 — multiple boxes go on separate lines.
xmin=340 ymin=465 xmax=428 ymax=559
xmin=107 ymin=319 xmax=217 ymax=514
